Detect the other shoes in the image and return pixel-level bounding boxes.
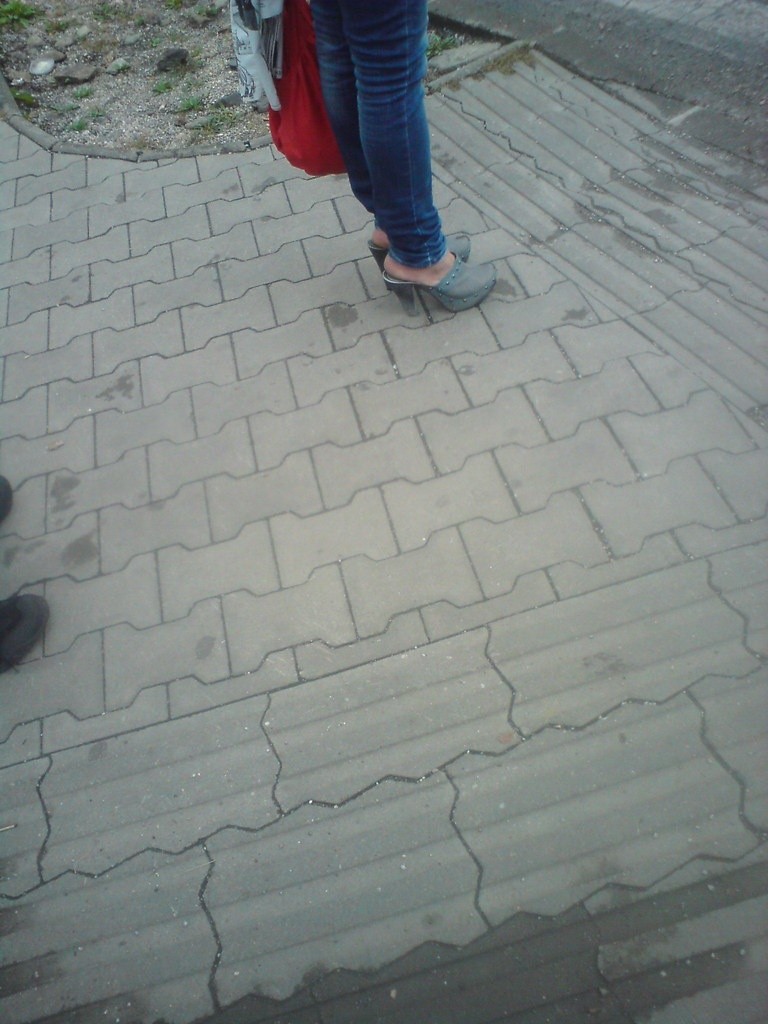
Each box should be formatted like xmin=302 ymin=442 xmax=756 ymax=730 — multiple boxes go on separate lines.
xmin=0 ymin=594 xmax=50 ymax=674
xmin=0 ymin=476 xmax=13 ymax=527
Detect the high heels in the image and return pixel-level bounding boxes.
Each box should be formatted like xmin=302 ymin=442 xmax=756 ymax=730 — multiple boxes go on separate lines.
xmin=381 ymin=249 xmax=497 ymax=317
xmin=368 ymin=235 xmax=471 ymax=275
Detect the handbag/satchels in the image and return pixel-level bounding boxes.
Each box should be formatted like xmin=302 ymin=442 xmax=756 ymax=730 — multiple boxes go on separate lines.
xmin=268 ymin=0 xmax=348 ymax=176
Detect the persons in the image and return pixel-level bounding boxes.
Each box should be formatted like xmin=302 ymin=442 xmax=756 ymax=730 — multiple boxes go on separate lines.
xmin=0 ymin=475 xmax=50 ymax=678
xmin=309 ymin=0 xmax=497 ymax=318
